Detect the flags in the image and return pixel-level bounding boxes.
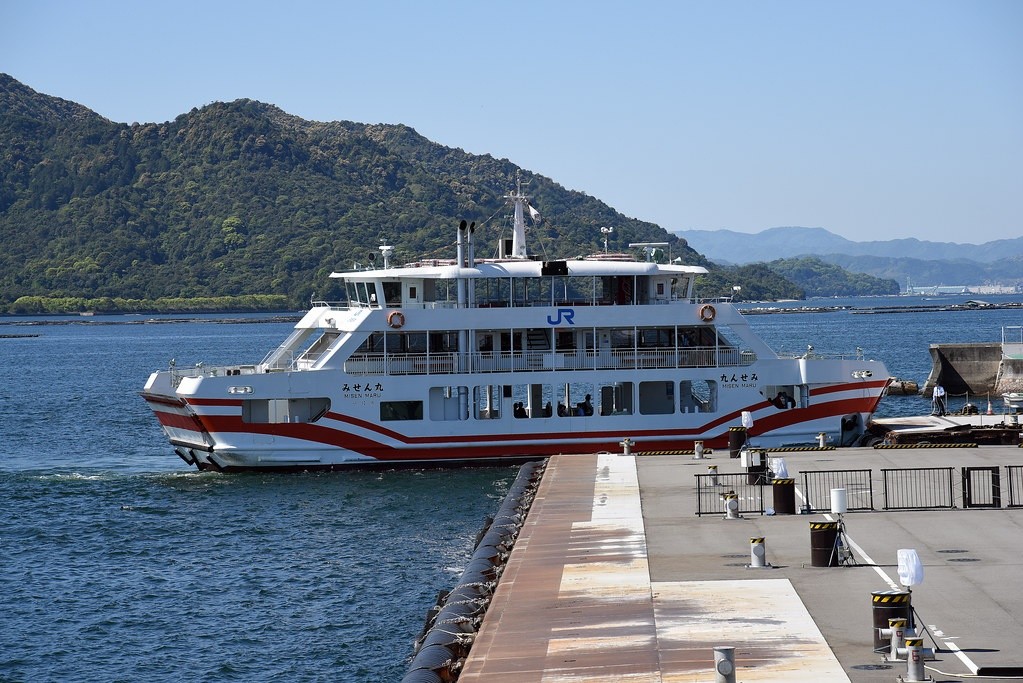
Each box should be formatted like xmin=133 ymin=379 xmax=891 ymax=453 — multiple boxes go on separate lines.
xmin=528 ymin=204 xmax=541 ymax=221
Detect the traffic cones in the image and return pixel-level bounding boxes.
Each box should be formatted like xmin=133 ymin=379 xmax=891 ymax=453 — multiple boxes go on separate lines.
xmin=985 ymin=400 xmax=994 ymax=415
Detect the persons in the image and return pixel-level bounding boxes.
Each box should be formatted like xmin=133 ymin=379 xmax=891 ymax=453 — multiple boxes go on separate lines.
xmin=385 ymin=285 xmax=401 ymax=307
xmin=385 ymin=403 xmax=400 ymax=419
xmin=584 ymin=394 xmax=593 ymax=413
xmin=773 ymin=392 xmax=796 ymax=409
xmin=686 ymin=331 xmax=697 ymax=364
xmin=514 ymin=402 xmax=528 ymax=418
xmin=576 ymin=403 xmax=584 ymax=416
xmin=933 ymin=381 xmax=945 ymax=412
xmin=558 ymin=404 xmax=567 ymax=416
xmin=672 ymin=330 xmax=684 ymax=347
xmin=542 ymin=405 xmax=551 ymax=417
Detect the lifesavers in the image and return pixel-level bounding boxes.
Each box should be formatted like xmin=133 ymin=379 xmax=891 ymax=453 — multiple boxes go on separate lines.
xmin=388 ymin=311 xmax=405 ymax=329
xmin=700 ymin=305 xmax=716 ymax=322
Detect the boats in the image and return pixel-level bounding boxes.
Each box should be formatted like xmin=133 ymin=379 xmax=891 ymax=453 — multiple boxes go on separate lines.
xmin=134 ymin=166 xmax=897 ymax=474
